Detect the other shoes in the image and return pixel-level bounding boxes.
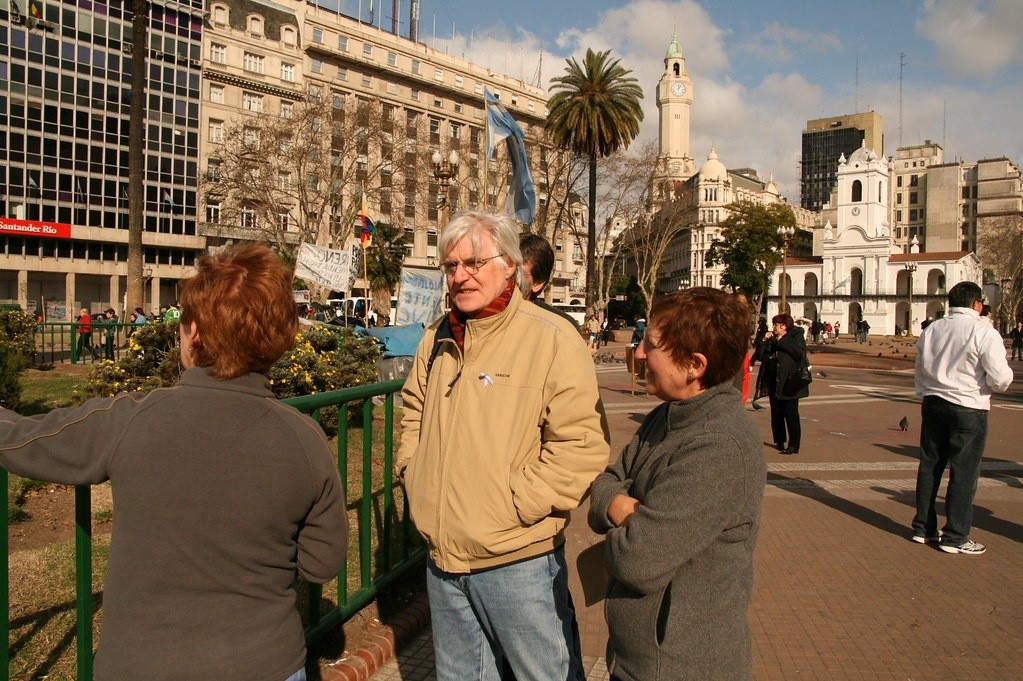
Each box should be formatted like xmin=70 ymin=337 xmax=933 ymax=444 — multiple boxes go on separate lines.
xmin=781 ymin=448 xmax=799 ymax=454
xmin=777 ymin=442 xmax=784 ymax=450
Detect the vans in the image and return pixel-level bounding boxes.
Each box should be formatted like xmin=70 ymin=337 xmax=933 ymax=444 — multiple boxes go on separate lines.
xmin=328 ymin=297 xmax=398 ymax=326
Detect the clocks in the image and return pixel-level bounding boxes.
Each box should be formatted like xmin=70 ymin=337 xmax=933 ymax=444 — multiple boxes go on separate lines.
xmin=850 ymin=206 xmax=861 ymax=216
xmin=670 ymin=81 xmax=687 ymax=97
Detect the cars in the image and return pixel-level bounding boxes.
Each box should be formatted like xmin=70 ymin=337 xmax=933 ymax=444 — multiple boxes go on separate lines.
xmin=76 ymin=314 xmax=116 ymax=328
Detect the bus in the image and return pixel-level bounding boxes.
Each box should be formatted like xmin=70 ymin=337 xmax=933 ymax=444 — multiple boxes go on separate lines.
xmin=554 ymin=304 xmax=586 ymax=325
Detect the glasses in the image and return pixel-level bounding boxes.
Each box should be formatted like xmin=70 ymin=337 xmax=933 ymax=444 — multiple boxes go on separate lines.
xmin=437 ymin=254 xmax=506 ymax=275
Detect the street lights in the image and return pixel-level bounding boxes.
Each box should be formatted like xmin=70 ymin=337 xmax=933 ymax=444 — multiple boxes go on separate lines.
xmin=431 ymin=149 xmax=460 ymax=236
xmin=905 ymin=262 xmax=918 ymax=336
xmin=141 ymin=266 xmax=152 ymax=316
xmin=1001 ymin=279 xmax=1012 ymax=335
xmin=776 ymin=226 xmax=796 ymax=314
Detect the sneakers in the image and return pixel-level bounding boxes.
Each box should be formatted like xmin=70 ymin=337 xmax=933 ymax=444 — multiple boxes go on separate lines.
xmin=939 ymin=539 xmax=987 ymax=555
xmin=913 ymin=530 xmax=943 ymax=544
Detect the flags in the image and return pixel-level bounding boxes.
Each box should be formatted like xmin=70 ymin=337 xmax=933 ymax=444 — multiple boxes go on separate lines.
xmin=357 ymin=191 xmax=377 ymax=250
xmin=484 ymin=85 xmax=536 ymax=225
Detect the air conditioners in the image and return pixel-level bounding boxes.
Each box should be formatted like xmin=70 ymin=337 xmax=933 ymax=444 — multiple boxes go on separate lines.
xmin=11 ymin=15 xmax=20 ymax=23
xmin=193 ymin=59 xmax=200 ymax=64
xmin=35 ymin=19 xmax=44 ymax=25
xmin=123 ymin=45 xmax=131 ymax=52
xmin=178 ymin=55 xmax=185 ymax=61
xmin=46 ymin=21 xmax=54 ymax=28
xmin=156 ymin=51 xmax=163 ymax=56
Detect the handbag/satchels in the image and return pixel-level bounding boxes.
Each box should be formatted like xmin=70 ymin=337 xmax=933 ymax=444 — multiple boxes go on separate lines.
xmin=800 ymin=369 xmax=812 ymax=384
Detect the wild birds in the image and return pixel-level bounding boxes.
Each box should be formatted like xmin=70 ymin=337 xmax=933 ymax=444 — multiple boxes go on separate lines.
xmin=593 ymin=351 xmax=627 ymax=365
xmin=855 ymin=335 xmax=918 ymax=358
xmin=819 ymin=370 xmax=827 ymax=377
xmin=820 ymin=340 xmax=836 ymax=345
xmin=899 ymin=416 xmax=910 ymax=432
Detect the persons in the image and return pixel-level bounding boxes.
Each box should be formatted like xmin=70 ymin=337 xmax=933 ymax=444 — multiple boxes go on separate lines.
xmin=600 ymin=317 xmax=612 ymax=346
xmin=74 ymin=300 xmax=182 ymax=362
xmin=586 ymin=315 xmax=600 ymax=350
xmin=1008 ymin=322 xmax=1023 ymax=362
xmin=810 ymin=318 xmax=871 ymax=345
xmin=588 ymin=287 xmax=767 ymax=681
xmin=395 ymin=210 xmax=611 ymax=681
xmin=630 ymin=312 xmax=646 ymax=379
xmin=980 ymin=304 xmax=994 ymax=326
xmin=295 ymin=300 xmax=353 ymax=324
xmin=912 ymin=281 xmax=1014 ymax=555
xmin=31 ymin=311 xmax=43 ymax=334
xmin=793 ymin=316 xmax=812 ymax=371
xmin=749 ymin=316 xmax=768 ymax=371
xmin=896 ymin=325 xmax=902 ymax=335
xmin=519 ymin=232 xmax=581 ymax=335
xmin=0 ymin=241 xmax=348 ymax=681
xmin=752 ymin=314 xmax=809 ymax=454
xmin=921 ymin=316 xmax=934 ymax=330
